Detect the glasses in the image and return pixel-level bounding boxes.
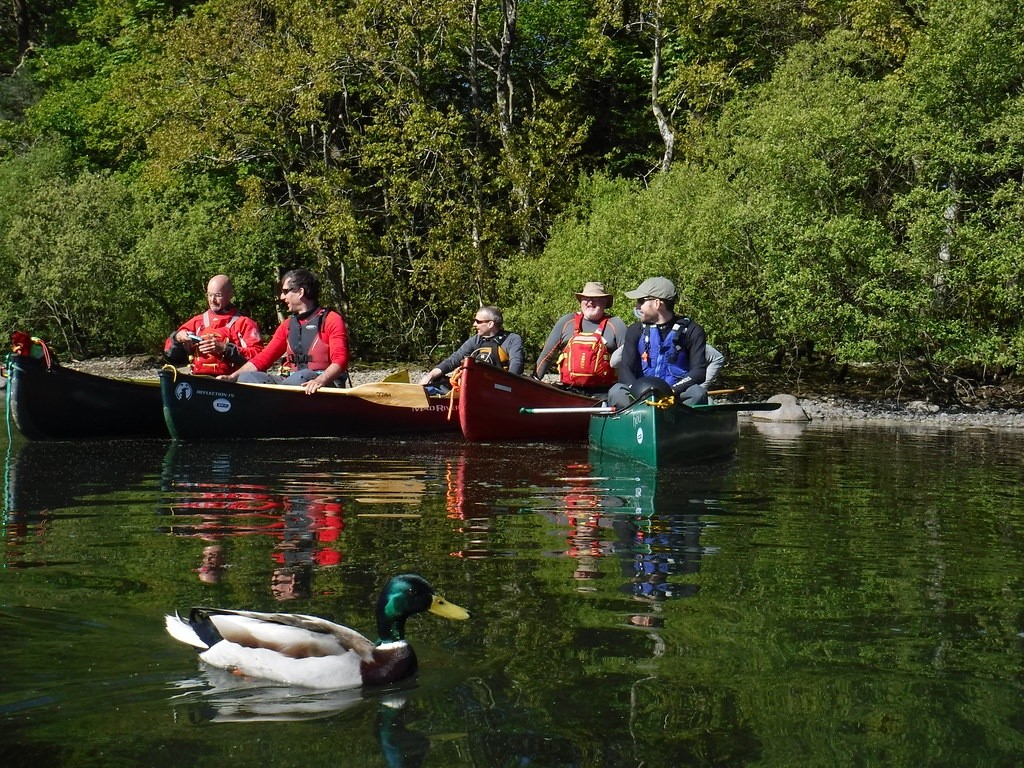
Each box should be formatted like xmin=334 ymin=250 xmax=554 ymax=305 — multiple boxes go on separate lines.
xmin=637 ymin=298 xmax=657 ymax=304
xmin=281 ymin=286 xmax=308 ymax=295
xmin=205 ymin=290 xmax=231 ymax=297
xmin=475 ymin=318 xmax=499 ymax=324
xmin=580 ymin=295 xmax=607 ymax=302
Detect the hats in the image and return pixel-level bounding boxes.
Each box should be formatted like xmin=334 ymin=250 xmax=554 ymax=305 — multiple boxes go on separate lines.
xmin=575 ymin=282 xmax=613 ymax=309
xmin=624 ymin=276 xmax=677 ymax=303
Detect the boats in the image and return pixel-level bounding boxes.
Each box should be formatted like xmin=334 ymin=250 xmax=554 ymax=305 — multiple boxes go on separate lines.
xmin=583 ymin=403 xmax=738 ymax=464
xmin=154 ymin=362 xmax=461 ymax=441
xmin=5 ymin=333 xmax=164 ymax=448
xmin=458 ymin=356 xmax=598 ymax=441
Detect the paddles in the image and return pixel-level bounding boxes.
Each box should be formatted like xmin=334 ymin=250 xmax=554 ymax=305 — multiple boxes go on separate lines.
xmin=705 ymin=384 xmax=748 ymax=396
xmin=428 ymin=388 xmax=461 ymax=399
xmin=218 ymin=379 xmax=429 ymax=411
xmin=516 ymin=402 xmax=785 ymax=414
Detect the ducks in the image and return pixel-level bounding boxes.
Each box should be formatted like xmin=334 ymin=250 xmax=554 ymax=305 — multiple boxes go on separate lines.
xmin=163 ymin=573 xmax=473 ymax=690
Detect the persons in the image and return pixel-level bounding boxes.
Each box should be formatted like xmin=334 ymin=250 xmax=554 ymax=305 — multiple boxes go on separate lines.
xmin=168 ymin=439 xmax=663 ymax=603
xmin=163 ymin=275 xmax=265 ymax=377
xmin=419 ymin=305 xmax=525 ymax=385
xmin=529 ymin=282 xmax=628 ymax=403
xmin=608 ymin=277 xmax=707 ymax=412
xmin=610 ymin=307 xmax=724 ymax=392
xmin=215 ymin=268 xmax=349 ymax=396
